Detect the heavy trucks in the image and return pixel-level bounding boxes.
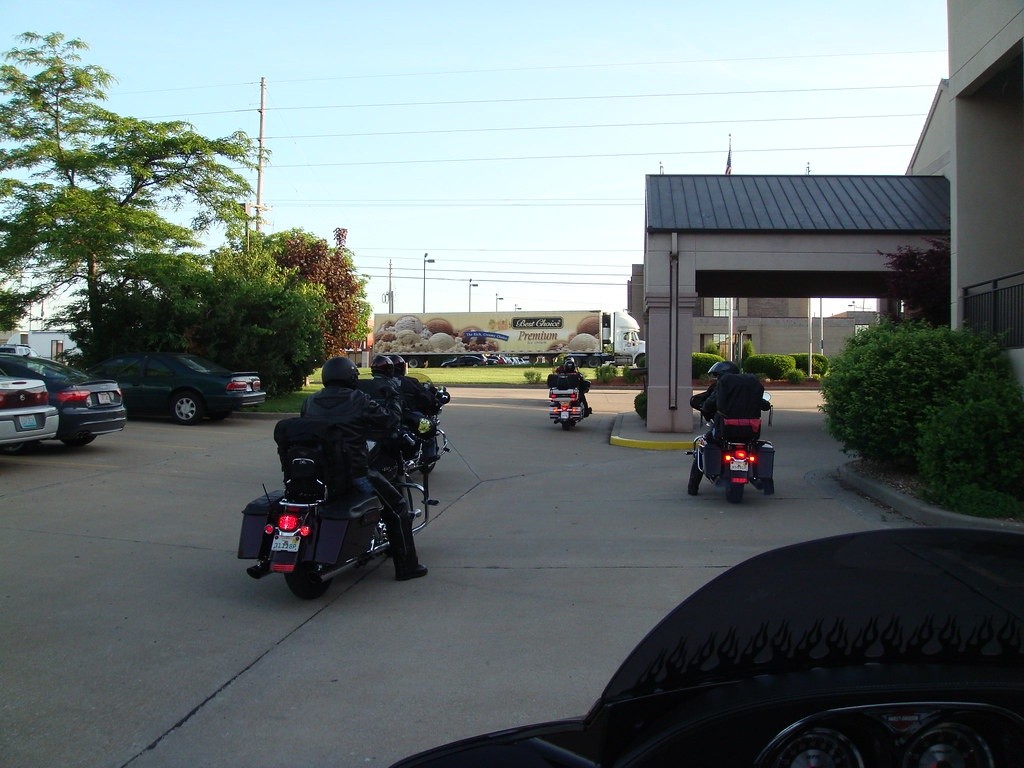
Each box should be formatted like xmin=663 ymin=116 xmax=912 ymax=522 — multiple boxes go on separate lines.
xmin=372 ymin=310 xmax=647 ymax=369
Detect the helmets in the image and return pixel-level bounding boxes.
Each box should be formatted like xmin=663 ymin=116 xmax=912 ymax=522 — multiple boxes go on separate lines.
xmin=707 ymin=361 xmax=739 ymax=377
xmin=389 ymin=354 xmax=406 ymax=376
xmin=564 ymin=356 xmax=575 ymax=364
xmin=322 ymin=356 xmax=360 ymax=386
xmin=370 ymin=355 xmax=394 ymax=377
xmin=564 ymin=361 xmax=576 ymax=372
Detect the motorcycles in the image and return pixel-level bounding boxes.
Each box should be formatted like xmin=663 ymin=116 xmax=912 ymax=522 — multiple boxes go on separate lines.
xmin=367 ymin=371 xmax=452 ymax=482
xmin=548 ymin=354 xmax=592 ymax=432
xmin=236 ymin=428 xmax=439 ymax=604
xmin=684 ymin=391 xmax=774 ymax=507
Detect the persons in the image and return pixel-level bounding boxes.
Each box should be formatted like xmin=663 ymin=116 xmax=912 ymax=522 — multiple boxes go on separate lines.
xmin=553 ymin=357 xmax=592 ymax=418
xmin=300 ymin=352 xmax=451 ymax=582
xmin=687 ymin=360 xmax=775 ymax=497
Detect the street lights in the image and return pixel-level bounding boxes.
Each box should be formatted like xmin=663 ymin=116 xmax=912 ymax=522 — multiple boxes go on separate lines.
xmin=423 ymin=259 xmax=435 ymax=314
xmin=515 ymin=308 xmax=522 ymax=311
xmin=496 ymin=297 xmax=503 ymax=312
xmin=848 ymin=305 xmax=855 ymax=311
xmin=469 ymin=284 xmax=478 ymax=311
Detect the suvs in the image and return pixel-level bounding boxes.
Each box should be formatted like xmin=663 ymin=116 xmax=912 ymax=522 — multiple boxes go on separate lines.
xmin=0 ymin=343 xmax=43 ymax=358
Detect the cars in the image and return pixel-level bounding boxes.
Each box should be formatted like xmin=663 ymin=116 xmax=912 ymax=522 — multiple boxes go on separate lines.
xmin=80 ymin=352 xmax=268 ymax=426
xmin=441 ymin=355 xmax=486 ymax=368
xmin=0 ymin=375 xmax=60 ymax=445
xmin=487 ymin=354 xmax=527 ymax=365
xmin=0 ymin=350 xmax=127 ymax=450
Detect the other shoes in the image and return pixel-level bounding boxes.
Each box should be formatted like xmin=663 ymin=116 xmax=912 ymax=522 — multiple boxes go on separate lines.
xmin=766 ymin=486 xmax=775 ymax=495
xmin=688 ymin=487 xmax=698 ymax=496
xmin=395 ymin=564 xmax=428 ymax=581
